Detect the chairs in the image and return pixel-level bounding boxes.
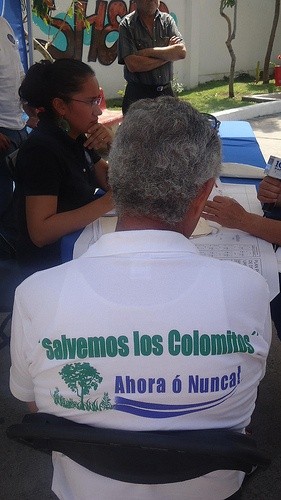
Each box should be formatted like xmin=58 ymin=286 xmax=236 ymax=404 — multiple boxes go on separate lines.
xmin=5 ymin=413 xmax=273 ymax=500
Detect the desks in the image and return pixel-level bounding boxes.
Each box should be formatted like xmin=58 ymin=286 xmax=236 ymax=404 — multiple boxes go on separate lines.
xmin=60 ymin=120 xmax=281 ymax=266
xmin=87 ymin=109 xmax=124 ymax=136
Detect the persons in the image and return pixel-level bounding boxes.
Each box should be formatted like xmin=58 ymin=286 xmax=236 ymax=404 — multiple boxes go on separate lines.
xmin=0 ymin=20 xmax=28 ymax=157
xmin=0 ymin=56 xmax=119 ymax=311
xmin=119 ymin=0 xmax=186 ymax=118
xmin=17 ymin=95 xmax=271 ymax=500
xmin=193 ymin=173 xmax=281 ymax=246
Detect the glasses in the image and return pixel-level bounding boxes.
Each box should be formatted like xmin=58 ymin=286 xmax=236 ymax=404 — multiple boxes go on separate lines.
xmin=70 ymin=97 xmax=102 ymax=108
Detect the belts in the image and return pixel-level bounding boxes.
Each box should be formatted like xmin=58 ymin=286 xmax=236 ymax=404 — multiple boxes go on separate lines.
xmin=138 ymin=84 xmax=171 ymax=92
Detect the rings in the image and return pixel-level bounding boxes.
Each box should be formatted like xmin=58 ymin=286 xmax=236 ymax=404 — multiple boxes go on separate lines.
xmin=204 ymin=214 xmax=209 ymax=220
xmin=262 ymin=190 xmax=266 ymax=196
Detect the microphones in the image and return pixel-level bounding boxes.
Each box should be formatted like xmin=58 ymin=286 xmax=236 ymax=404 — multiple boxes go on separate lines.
xmin=262 ymin=155 xmax=281 ymax=213
